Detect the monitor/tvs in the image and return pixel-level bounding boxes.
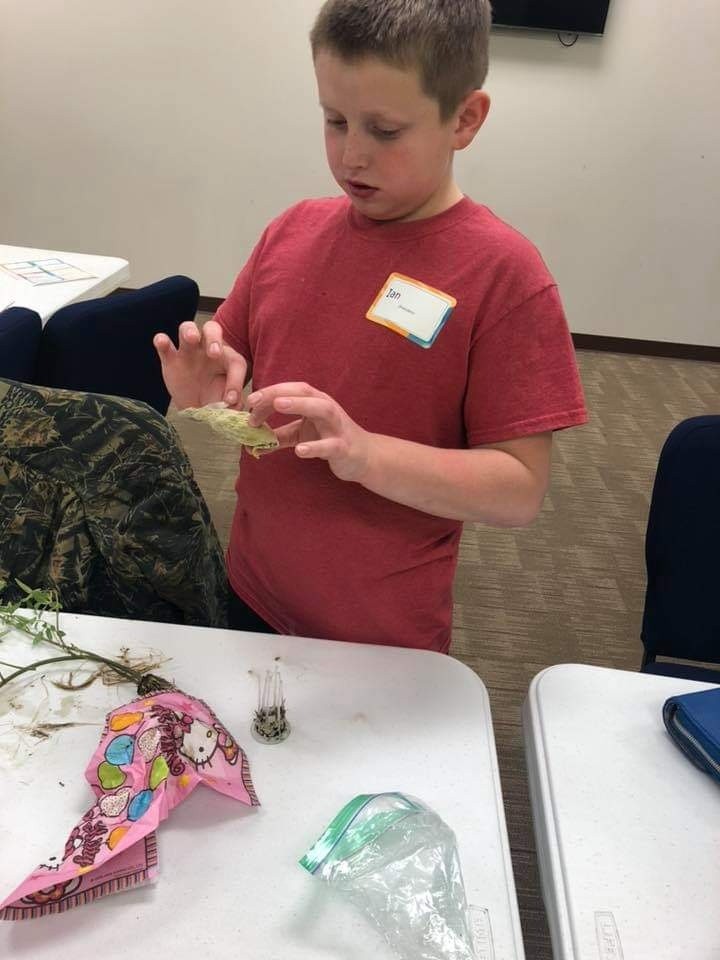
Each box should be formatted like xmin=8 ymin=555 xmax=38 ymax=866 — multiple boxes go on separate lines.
xmin=488 ymin=0 xmax=611 ymax=36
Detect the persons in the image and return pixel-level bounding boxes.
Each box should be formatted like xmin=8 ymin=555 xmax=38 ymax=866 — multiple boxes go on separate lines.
xmin=154 ymin=0 xmax=590 ymax=655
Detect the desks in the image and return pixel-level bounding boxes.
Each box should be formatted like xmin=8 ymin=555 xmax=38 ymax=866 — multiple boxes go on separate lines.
xmin=0 ymin=606 xmax=525 ymax=960
xmin=524 ymin=661 xmax=720 ymax=960
xmin=0 ymin=244 xmax=130 ymax=324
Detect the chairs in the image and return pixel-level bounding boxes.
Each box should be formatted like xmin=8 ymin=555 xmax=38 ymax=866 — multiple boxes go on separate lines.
xmin=0 ymin=378 xmax=226 ymax=632
xmin=639 ymin=414 xmax=720 ymax=685
xmin=42 ymin=276 xmax=201 ymax=419
xmin=0 ymin=307 xmax=42 ymax=386
